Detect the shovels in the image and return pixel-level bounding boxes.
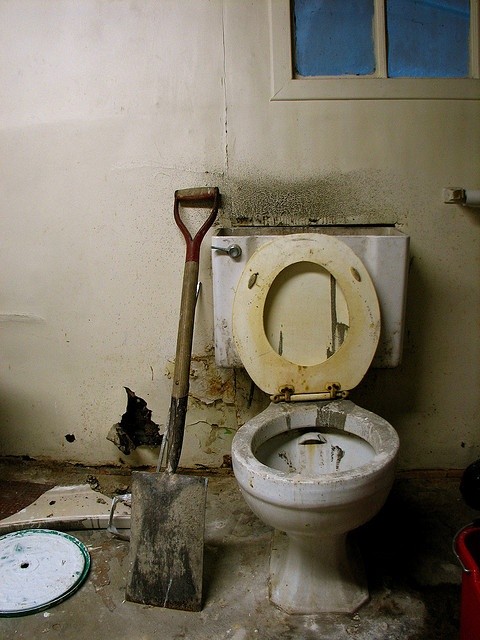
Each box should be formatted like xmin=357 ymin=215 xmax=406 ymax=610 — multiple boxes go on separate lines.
xmin=122 ymin=186 xmax=221 ymax=612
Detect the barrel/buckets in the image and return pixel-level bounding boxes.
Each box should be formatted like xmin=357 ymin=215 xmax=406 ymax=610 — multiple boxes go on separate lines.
xmin=449 ymin=521 xmax=480 ymax=640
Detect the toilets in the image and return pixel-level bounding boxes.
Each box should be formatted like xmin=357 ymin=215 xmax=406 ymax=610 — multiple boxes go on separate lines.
xmin=209 ymin=224 xmax=410 ymax=612
